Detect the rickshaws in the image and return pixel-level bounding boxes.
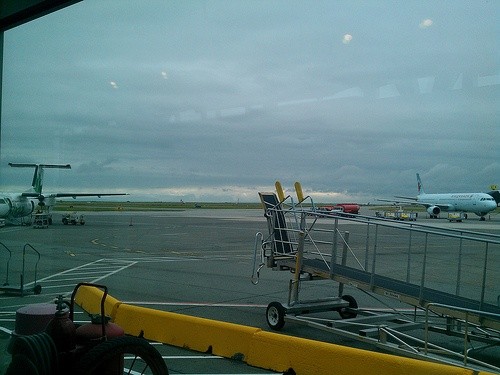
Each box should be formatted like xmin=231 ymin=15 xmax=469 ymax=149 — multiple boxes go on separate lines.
xmin=31 ymin=212 xmax=52 ymax=230
xmin=1 ymin=241 xmax=42 ymax=296
xmin=249 ymin=188 xmax=500 ymax=375
xmin=62 ymin=211 xmax=85 ymax=226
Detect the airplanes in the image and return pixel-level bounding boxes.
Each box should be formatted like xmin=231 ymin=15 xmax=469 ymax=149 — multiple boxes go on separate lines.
xmin=0 ymin=162 xmax=130 ymax=233
xmin=374 ymin=171 xmax=500 ymax=221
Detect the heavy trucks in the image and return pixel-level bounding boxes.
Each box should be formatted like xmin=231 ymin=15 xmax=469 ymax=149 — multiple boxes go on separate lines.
xmin=310 ymin=202 xmax=361 ymax=217
